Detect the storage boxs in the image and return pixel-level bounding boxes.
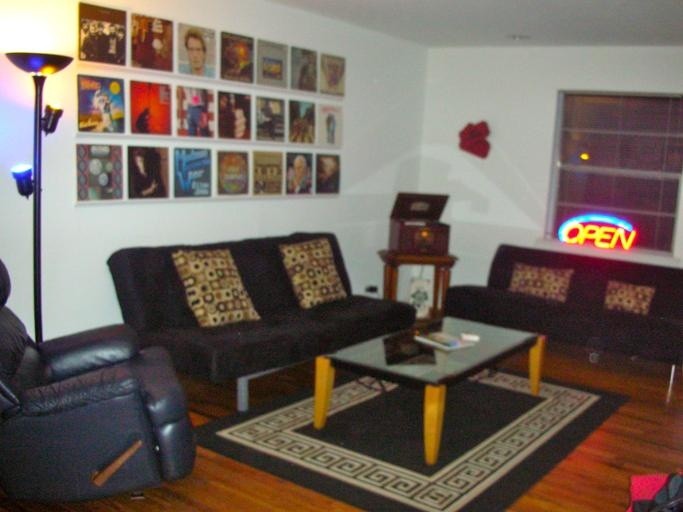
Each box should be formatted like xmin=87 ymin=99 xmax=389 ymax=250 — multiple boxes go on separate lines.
xmin=389 ymin=191 xmax=450 ymax=257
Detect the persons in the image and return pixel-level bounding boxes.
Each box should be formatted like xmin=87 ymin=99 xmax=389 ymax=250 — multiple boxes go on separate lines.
xmin=80 ymin=19 xmax=125 ymax=65
xmin=218 ymin=92 xmax=247 ymax=139
xmin=177 ymin=86 xmax=212 ymax=137
xmin=128 ymin=147 xmax=167 ymax=198
xmin=287 ymin=155 xmax=311 ymax=194
xmin=179 ymin=28 xmax=215 ymax=77
xmin=318 ymin=157 xmax=338 ymax=192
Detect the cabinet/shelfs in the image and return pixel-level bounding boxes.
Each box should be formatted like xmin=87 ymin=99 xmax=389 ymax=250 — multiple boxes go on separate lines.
xmin=380 ymin=251 xmax=458 ymax=315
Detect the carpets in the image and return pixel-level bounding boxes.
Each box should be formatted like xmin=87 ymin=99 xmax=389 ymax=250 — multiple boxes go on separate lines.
xmin=191 ymin=371 xmax=630 ymax=512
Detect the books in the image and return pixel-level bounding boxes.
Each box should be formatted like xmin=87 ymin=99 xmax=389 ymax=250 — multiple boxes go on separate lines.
xmin=414 ymin=331 xmax=474 ymax=351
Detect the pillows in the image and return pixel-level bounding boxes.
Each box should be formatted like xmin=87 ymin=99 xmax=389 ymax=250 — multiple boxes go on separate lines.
xmin=507 ymin=259 xmax=575 ymax=303
xmin=603 ymin=279 xmax=655 ymax=315
xmin=278 ymin=237 xmax=348 ymax=311
xmin=170 ymin=246 xmax=261 ymax=329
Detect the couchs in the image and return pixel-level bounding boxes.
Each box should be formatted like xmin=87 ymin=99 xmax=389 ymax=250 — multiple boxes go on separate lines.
xmin=444 ymin=243 xmax=683 ymax=391
xmin=107 ymin=230 xmax=420 ymax=414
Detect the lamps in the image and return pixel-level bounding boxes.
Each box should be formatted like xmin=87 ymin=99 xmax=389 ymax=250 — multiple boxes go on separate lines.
xmin=5 ymin=52 xmax=74 ymax=343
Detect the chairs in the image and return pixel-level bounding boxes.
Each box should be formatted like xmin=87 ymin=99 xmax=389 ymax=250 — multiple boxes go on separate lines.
xmin=0 ymin=259 xmax=194 ymax=504
